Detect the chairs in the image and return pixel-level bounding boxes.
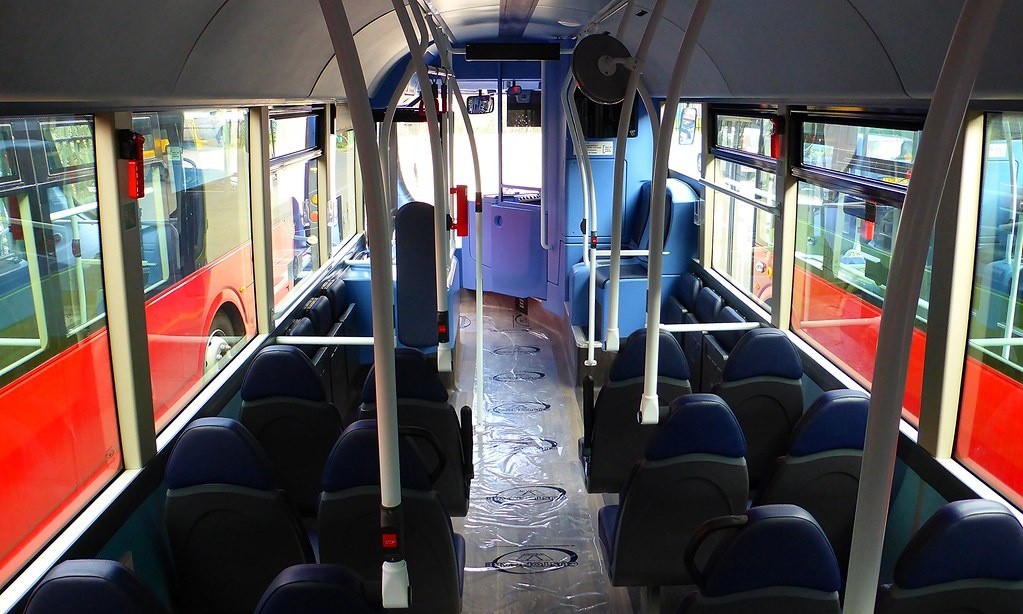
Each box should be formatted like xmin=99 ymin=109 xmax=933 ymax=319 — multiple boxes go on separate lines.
xmin=564 ymin=179 xmax=1023 ymax=614
xmin=0 ymin=159 xmax=475 ymax=614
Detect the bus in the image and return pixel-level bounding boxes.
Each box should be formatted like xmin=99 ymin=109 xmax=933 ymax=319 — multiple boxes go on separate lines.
xmin=0 ymin=114 xmax=306 ymax=596
xmin=749 ymin=118 xmax=1023 ymax=517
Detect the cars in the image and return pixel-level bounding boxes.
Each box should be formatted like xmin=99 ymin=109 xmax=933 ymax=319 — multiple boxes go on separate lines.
xmin=183 ymin=110 xmax=244 ymax=144
